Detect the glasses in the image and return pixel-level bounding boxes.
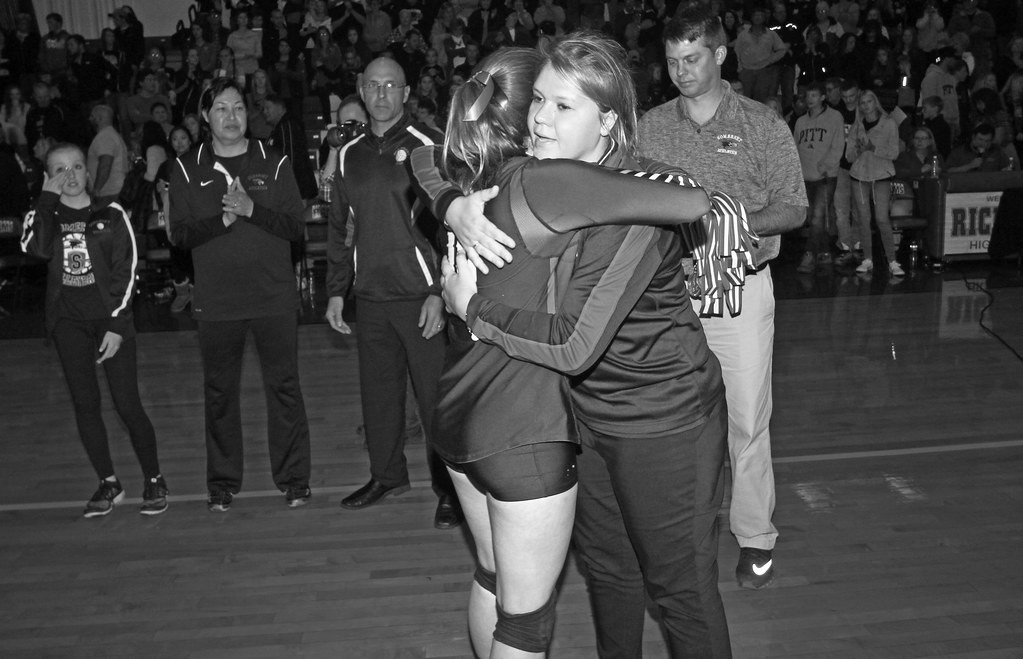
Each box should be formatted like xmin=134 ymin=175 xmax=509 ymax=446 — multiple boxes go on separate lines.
xmin=150 ymin=53 xmax=159 ymax=58
xmin=914 ymin=136 xmax=929 ymax=140
xmin=361 ymin=81 xmax=406 ymax=92
xmin=210 ymin=14 xmax=221 ymax=18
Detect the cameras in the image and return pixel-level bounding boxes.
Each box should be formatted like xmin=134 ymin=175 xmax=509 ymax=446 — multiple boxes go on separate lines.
xmin=327 ymin=120 xmax=367 ymax=148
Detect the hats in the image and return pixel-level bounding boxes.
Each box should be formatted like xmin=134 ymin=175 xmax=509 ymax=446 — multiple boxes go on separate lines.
xmin=107 ymin=8 xmax=122 ymax=16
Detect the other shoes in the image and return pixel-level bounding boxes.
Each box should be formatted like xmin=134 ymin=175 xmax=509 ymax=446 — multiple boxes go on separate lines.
xmin=207 ymin=488 xmax=233 ymax=512
xmin=855 ymin=259 xmax=873 ymax=273
xmin=286 ymin=484 xmax=311 ymax=507
xmin=853 ymin=252 xmax=864 ymax=265
xmin=798 ymin=252 xmax=816 ymax=274
xmin=888 ymin=261 xmax=905 ymax=275
xmin=835 ymin=252 xmax=852 ymax=266
xmin=817 ymin=253 xmax=833 ymax=264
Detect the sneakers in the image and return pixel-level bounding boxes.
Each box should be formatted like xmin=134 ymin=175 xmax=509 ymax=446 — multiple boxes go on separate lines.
xmin=84 ymin=478 xmax=125 ymax=518
xmin=139 ymin=473 xmax=169 ymax=515
xmin=736 ymin=547 xmax=776 ymax=590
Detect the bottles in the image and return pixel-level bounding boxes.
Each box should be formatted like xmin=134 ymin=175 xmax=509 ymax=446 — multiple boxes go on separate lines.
xmin=931 ymin=155 xmax=938 ymax=176
xmin=1009 ymin=157 xmax=1014 ymax=172
xmin=910 ymin=241 xmax=918 ymax=270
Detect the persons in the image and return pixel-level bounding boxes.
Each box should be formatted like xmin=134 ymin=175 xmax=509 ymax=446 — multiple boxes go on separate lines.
xmin=0 ymin=0 xmax=1023 ymax=659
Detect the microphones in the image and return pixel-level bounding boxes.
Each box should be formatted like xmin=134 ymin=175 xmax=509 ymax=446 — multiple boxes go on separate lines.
xmin=974 ymin=148 xmax=984 ymax=172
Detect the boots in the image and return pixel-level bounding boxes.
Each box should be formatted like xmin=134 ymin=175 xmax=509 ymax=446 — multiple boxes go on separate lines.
xmin=170 ymin=276 xmax=190 ymax=312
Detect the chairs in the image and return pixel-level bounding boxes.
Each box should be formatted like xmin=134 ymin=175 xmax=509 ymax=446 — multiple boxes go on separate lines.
xmin=301 ymin=206 xmax=331 ymax=306
xmin=876 ymin=182 xmax=929 ymax=269
xmin=0 ymin=217 xmax=31 ymax=318
xmin=143 ymin=212 xmax=177 ymax=312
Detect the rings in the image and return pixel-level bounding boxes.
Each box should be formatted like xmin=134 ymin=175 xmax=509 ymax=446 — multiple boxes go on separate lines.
xmin=232 ymin=203 xmax=236 ymax=208
xmin=438 ymin=325 xmax=442 ymax=328
xmin=472 ymin=241 xmax=478 ymax=249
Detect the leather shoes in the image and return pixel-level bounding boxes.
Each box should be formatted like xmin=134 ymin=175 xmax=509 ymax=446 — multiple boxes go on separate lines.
xmin=340 ymin=478 xmax=410 ymax=510
xmin=434 ymin=496 xmax=464 ymax=529
